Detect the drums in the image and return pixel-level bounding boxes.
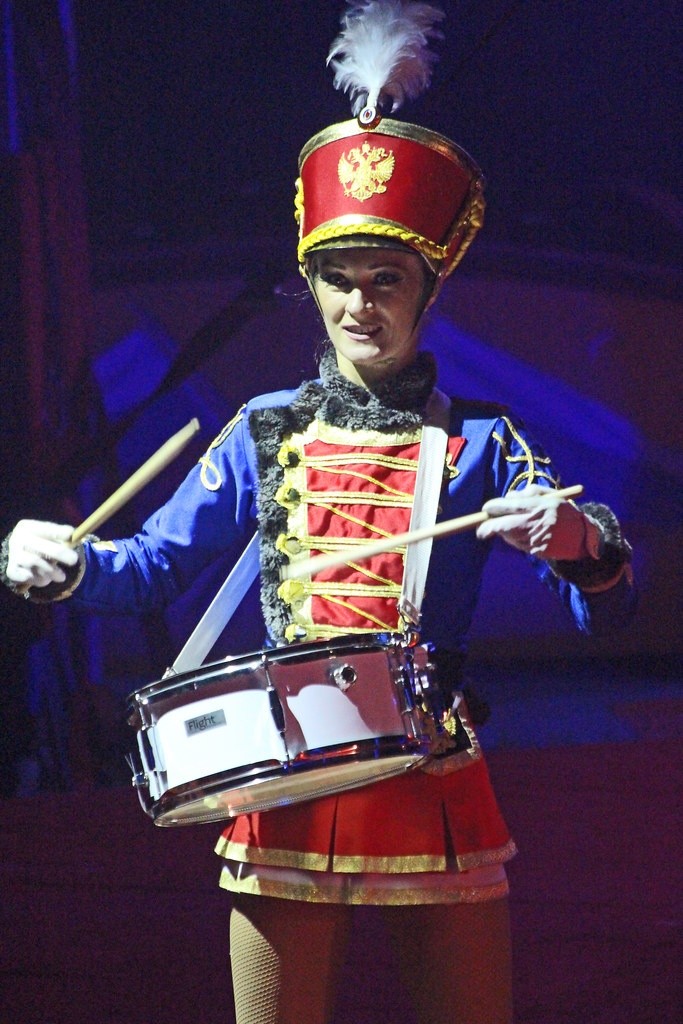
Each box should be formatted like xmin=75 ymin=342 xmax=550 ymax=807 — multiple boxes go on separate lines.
xmin=122 ymin=630 xmax=435 ymax=830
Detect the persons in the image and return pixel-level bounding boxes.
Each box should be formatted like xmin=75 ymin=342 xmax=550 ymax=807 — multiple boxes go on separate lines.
xmin=0 ymin=0 xmax=682 ymax=1024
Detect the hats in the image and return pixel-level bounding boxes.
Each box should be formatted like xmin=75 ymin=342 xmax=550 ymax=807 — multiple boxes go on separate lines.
xmin=295 ymin=0 xmax=487 ymax=280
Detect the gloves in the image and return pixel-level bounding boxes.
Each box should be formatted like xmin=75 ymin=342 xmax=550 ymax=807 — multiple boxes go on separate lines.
xmin=6 ymin=519 xmax=86 ymax=587
xmin=477 ymin=484 xmax=585 ymax=559
